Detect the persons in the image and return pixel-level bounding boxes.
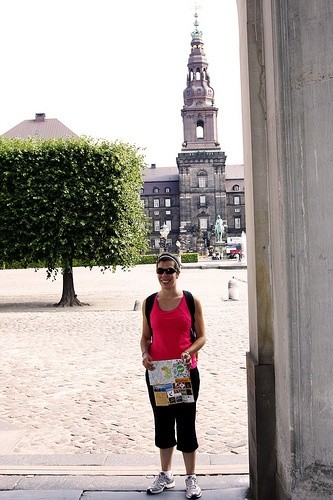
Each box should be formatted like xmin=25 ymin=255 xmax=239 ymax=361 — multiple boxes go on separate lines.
xmin=139 ymin=253 xmax=206 ymax=500
xmin=216 ymin=215 xmax=224 ymax=235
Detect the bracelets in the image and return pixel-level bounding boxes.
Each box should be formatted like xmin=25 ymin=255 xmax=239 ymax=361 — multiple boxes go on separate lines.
xmin=142 ymin=351 xmax=148 ymax=356
xmin=184 ymin=351 xmax=190 ymax=355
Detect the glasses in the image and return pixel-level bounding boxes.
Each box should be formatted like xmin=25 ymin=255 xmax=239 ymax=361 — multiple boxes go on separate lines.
xmin=155 ymin=266 xmax=179 ymax=275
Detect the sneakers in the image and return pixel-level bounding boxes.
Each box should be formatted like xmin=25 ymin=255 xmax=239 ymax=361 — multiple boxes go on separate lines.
xmin=146 ymin=471 xmax=202 ymax=499
